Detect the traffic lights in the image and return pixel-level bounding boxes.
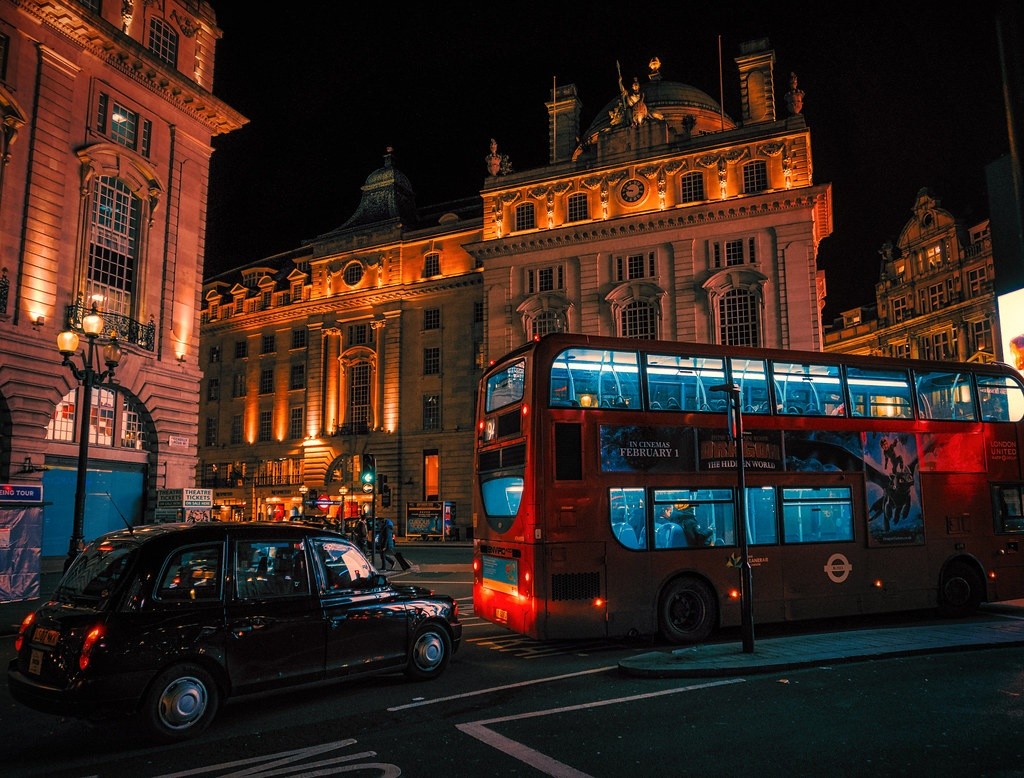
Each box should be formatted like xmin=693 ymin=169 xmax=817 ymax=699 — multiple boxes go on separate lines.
xmin=363 ymin=453 xmax=376 ymax=493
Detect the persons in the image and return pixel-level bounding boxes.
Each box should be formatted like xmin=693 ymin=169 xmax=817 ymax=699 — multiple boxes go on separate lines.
xmin=485 ymin=139 xmax=502 ymax=177
xmin=880 ymin=438 xmax=903 ymax=474
xmin=618 ymin=77 xmax=654 ymax=125
xmin=317 ymin=544 xmax=348 ymax=590
xmin=655 ymin=505 xmax=671 ymax=525
xmin=374 ymin=519 xmax=396 ymax=572
xmin=358 ymin=515 xmax=367 ymax=557
xmin=898 ymin=407 xmax=911 ymax=418
xmin=670 ymin=498 xmax=713 ymax=548
xmin=783 ymin=72 xmax=804 ymax=114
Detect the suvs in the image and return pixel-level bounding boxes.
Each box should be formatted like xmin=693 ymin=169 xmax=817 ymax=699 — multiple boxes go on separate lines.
xmin=288 ymin=516 xmax=395 ymax=552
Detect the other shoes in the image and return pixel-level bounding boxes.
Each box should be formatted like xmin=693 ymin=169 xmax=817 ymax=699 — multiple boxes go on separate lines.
xmin=391 ymin=561 xmax=396 ymax=570
xmin=379 ymin=569 xmax=386 ymax=572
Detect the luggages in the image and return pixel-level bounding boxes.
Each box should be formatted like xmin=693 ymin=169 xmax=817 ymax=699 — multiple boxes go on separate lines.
xmin=390 ymin=549 xmax=412 ymax=571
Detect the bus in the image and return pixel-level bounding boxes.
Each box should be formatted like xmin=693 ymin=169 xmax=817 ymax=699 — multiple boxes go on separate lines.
xmin=471 ymin=332 xmax=1024 ymax=648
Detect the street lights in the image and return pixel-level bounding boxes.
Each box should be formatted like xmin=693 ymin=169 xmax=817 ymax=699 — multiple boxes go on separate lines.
xmin=707 ymin=383 xmax=754 ymax=655
xmin=56 ymin=301 xmax=123 ymax=576
xmin=338 ymin=486 xmax=348 ymax=535
xmin=299 ymin=485 xmax=309 ymax=516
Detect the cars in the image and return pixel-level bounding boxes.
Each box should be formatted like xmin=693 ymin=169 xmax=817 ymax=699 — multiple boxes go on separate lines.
xmin=7 ymin=521 xmax=465 ymax=745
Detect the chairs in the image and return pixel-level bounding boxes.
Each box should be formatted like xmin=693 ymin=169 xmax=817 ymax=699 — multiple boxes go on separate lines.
xmin=700 ymin=404 xmax=712 ymax=411
xmin=761 ymin=400 xmax=769 ymax=412
xmin=715 ymin=536 xmax=726 ymax=545
xmin=639 ymin=520 xmax=666 ymax=547
xmin=613 ymin=395 xmax=628 ymax=408
xmin=744 ymin=404 xmax=755 ymax=412
xmin=658 ymin=522 xmax=687 ymax=548
xmin=600 ymin=399 xmax=611 ymax=407
xmin=651 ymin=400 xmax=661 ymax=409
xmin=612 ymin=522 xmax=638 ymax=549
xmin=805 ymin=403 xmax=820 ymax=414
xmin=666 ymin=397 xmax=680 ymax=409
xmin=716 ymin=399 xmax=727 ymax=411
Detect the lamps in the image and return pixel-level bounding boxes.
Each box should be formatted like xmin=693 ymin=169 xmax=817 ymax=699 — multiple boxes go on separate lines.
xmin=31 ymin=316 xmax=45 ymax=327
xmin=176 ymin=354 xmax=186 ymax=363
